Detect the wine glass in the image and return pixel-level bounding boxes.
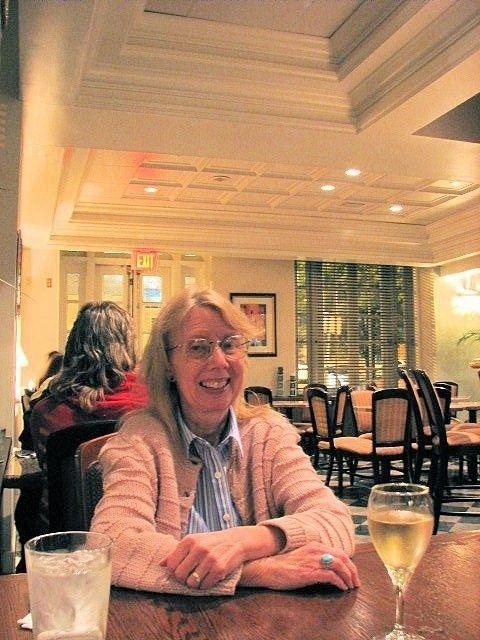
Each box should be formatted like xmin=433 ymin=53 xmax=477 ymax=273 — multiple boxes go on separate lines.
xmin=191 ymin=572 xmax=203 ymax=584
xmin=319 ymin=552 xmax=333 ymax=570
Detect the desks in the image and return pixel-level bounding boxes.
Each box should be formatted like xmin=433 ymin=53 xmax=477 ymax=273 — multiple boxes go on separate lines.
xmin=272 ymin=400 xmax=310 ymax=427
xmin=0 ymin=530 xmax=480 ymax=640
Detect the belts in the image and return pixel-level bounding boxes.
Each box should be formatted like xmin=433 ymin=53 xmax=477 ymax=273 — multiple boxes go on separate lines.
xmin=364 ymin=482 xmax=434 ymax=640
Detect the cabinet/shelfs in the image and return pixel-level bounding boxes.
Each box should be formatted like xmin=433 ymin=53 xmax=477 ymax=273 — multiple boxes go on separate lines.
xmin=289 ymin=375 xmax=298 ymax=397
xmin=277 ymin=367 xmax=284 ymax=397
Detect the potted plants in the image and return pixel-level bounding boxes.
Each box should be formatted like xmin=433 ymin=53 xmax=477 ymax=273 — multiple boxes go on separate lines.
xmin=457 ymin=329 xmax=480 ymax=379
xmin=229 ymin=292 xmax=277 ymax=357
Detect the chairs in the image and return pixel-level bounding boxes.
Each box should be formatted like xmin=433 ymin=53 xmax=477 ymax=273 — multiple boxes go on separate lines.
xmin=21 ymin=387 xmax=118 ymax=541
xmin=244 ymin=386 xmax=273 ymax=410
xmin=410 ymin=368 xmax=479 ymax=534
xmin=293 ymin=383 xmax=328 ymax=454
xmin=306 ymin=365 xmax=480 ymax=500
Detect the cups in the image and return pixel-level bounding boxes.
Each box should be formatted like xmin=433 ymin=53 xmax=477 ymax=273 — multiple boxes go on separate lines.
xmin=166 ymin=335 xmax=251 ymax=360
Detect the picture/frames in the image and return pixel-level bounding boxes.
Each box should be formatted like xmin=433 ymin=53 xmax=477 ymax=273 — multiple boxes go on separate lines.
xmin=0 ymin=530 xmax=480 ymax=640
xmin=229 ymin=292 xmax=277 ymax=357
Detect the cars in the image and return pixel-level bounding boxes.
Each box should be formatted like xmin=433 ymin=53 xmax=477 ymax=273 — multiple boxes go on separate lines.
xmin=24 ymin=529 xmax=116 ymax=639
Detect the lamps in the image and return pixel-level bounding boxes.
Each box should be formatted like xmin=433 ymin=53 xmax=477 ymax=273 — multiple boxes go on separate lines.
xmin=21 ymin=387 xmax=118 ymax=541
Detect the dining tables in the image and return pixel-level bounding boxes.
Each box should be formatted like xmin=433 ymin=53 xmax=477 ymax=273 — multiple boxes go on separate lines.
xmin=457 ymin=329 xmax=480 ymax=379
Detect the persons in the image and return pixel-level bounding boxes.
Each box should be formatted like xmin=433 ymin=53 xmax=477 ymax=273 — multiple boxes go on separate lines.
xmin=31 ymin=351 xmax=66 ymax=387
xmin=84 ymin=286 xmax=362 ymax=597
xmin=29 ymin=301 xmax=150 ymax=458
